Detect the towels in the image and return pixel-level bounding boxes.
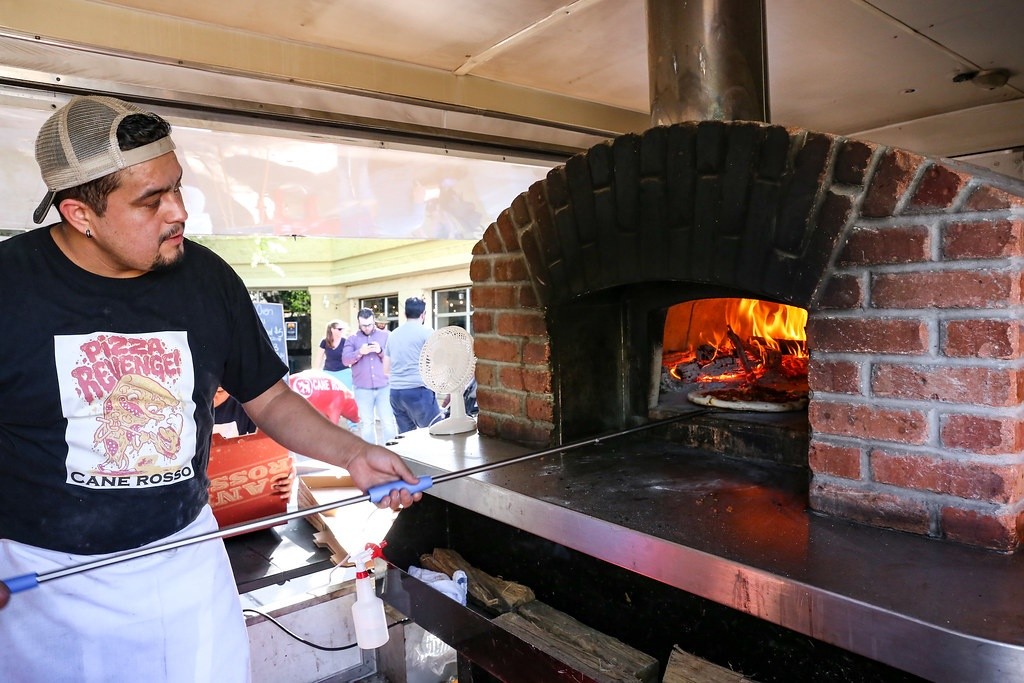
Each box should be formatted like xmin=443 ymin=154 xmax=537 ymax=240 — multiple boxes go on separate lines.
xmin=406 ymin=566 xmax=468 ymax=606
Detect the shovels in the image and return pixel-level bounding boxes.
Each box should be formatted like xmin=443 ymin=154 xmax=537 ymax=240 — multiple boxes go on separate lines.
xmin=1 ymin=381 xmax=796 ymax=594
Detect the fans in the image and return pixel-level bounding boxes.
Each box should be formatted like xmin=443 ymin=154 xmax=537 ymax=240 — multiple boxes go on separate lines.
xmin=419 ymin=324 xmax=479 ymax=436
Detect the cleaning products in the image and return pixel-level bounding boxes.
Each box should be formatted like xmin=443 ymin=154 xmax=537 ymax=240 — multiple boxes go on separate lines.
xmin=347 ymin=541 xmax=389 ymax=650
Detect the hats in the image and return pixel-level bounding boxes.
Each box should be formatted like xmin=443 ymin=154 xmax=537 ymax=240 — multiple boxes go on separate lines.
xmin=33 ymin=94 xmax=177 ymax=224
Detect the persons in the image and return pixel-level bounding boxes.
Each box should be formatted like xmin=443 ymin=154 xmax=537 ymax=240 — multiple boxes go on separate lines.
xmin=0 ymin=96 xmax=423 ymax=683
xmin=211 ymin=383 xmax=255 ymax=435
xmin=317 ymin=297 xmax=442 ymax=446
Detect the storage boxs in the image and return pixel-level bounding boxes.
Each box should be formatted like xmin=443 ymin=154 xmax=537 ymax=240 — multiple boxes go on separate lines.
xmin=206 ymin=426 xmax=292 ymax=538
xmin=296 ymin=475 xmax=405 ymax=568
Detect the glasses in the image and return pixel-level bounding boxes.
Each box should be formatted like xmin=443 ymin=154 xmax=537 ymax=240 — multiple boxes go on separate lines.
xmin=333 ymin=328 xmax=343 ymax=331
